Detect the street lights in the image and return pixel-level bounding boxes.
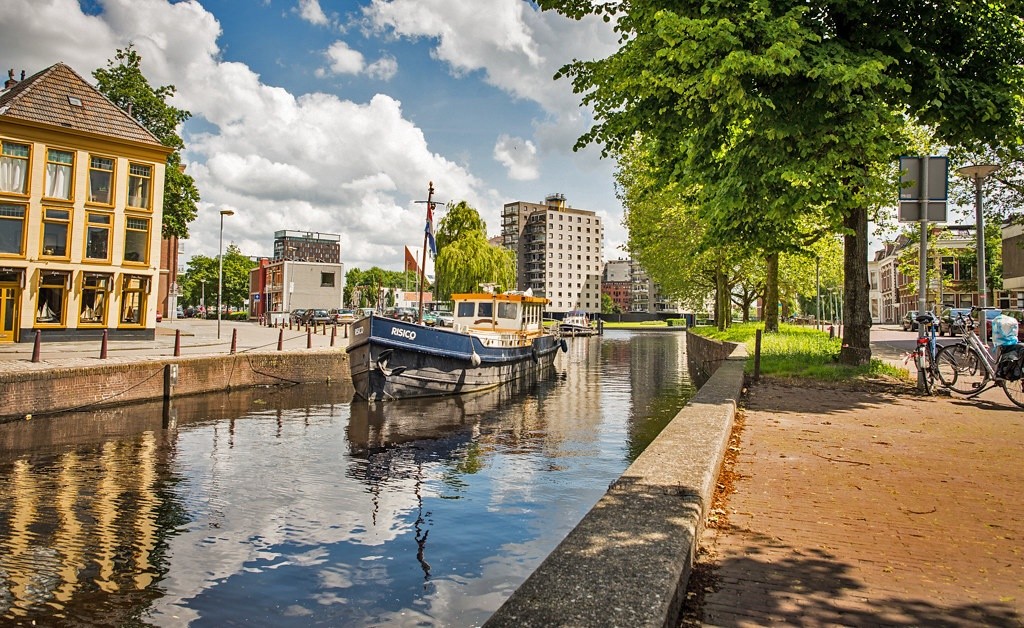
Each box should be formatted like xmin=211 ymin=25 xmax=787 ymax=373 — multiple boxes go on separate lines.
xmin=199 ymin=276 xmax=206 ymax=306
xmin=813 ymin=255 xmax=823 ymax=331
xmin=956 ymin=164 xmax=1002 ymax=376
xmin=820 ymin=285 xmax=844 ymax=327
xmin=216 ymin=210 xmax=235 ymax=340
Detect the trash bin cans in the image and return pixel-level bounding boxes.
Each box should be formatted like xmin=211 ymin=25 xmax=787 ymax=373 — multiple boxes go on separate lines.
xmin=267 ymin=312 xmax=290 ymax=328
xmin=686 ymin=314 xmax=695 ymax=327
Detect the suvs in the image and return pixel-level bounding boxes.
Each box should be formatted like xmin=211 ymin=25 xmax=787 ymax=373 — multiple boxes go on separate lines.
xmin=938 ymin=306 xmax=972 ymax=338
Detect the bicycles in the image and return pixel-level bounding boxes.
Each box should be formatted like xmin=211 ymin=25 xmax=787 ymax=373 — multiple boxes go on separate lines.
xmin=914 ymin=304 xmax=958 ymax=395
xmin=934 ymin=305 xmax=1024 ymax=407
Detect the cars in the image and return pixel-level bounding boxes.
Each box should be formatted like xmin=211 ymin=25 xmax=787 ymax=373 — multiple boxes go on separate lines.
xmin=902 ymin=310 xmax=940 ymax=332
xmin=964 ymin=306 xmax=1004 ymax=341
xmin=289 ymin=309 xmax=307 ymax=324
xmin=177 ymin=308 xmax=185 ymax=319
xmin=1001 ymin=308 xmax=1024 ymax=343
xmin=299 ymin=309 xmax=334 ymax=326
xmin=184 ymin=307 xmax=196 ymax=318
xmin=329 ymin=307 xmax=355 ymax=323
xmin=349 ymin=307 xmax=454 ymax=327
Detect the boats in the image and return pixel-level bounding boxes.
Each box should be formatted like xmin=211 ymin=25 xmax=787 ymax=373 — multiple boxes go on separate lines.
xmin=558 ymin=310 xmax=594 ymax=337
xmin=345 ymin=181 xmax=569 ymax=406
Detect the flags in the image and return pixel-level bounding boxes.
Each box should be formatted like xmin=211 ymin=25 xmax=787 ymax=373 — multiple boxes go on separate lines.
xmin=425 ymin=202 xmax=438 ymax=257
